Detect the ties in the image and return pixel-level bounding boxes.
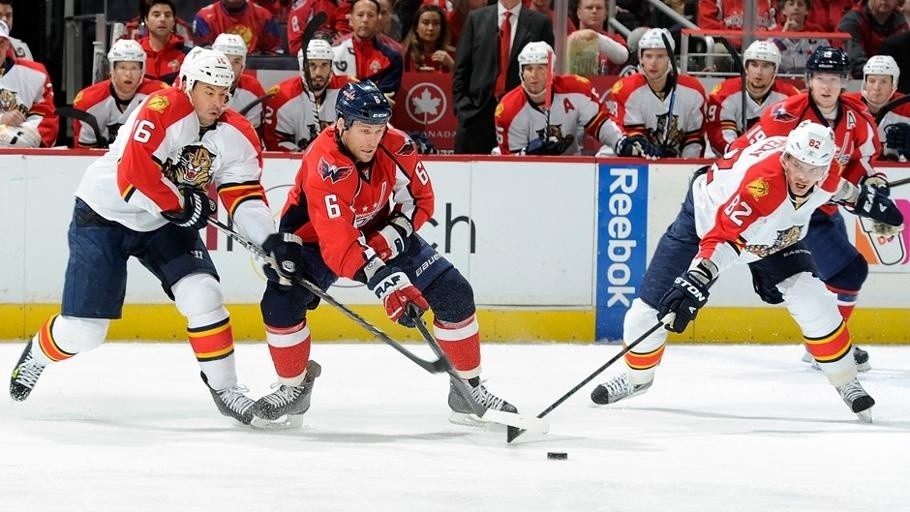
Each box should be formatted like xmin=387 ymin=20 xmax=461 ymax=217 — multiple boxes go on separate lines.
xmin=493 ymin=12 xmax=511 ymax=98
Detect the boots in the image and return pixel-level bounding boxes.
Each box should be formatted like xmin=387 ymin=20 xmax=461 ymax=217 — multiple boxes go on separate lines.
xmin=801 ymin=351 xmax=812 ymax=363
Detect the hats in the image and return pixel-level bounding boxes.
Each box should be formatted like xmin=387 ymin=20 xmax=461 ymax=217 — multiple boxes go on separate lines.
xmin=625 ymin=26 xmax=650 ymax=55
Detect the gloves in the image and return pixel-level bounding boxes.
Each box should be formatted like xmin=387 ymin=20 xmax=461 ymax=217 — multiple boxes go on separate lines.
xmin=653 ymin=271 xmax=712 ymax=336
xmin=366 ymin=264 xmax=430 ymax=328
xmin=850 ymin=183 xmax=906 ymax=237
xmin=260 ymin=231 xmax=305 ymax=290
xmin=612 ymin=133 xmax=661 ymax=160
xmin=364 ymin=212 xmax=414 ymax=260
xmin=885 ymin=117 xmax=910 ymax=160
xmin=863 ymin=174 xmax=890 ymax=197
xmin=162 ymin=185 xmax=209 ymax=230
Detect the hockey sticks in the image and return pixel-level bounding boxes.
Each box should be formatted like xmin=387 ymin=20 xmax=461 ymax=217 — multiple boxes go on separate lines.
xmin=206 ymin=217 xmax=451 ymax=375
xmin=240 ymin=93 xmax=275 ymax=114
xmin=407 ymin=300 xmax=549 ymax=434
xmin=54 ymin=107 xmax=105 ymax=148
xmin=721 ymin=36 xmax=747 ymax=136
xmin=299 ymin=11 xmax=327 ymax=135
xmin=662 ymin=33 xmax=678 ymax=144
xmin=506 ymin=316 xmax=674 ymax=445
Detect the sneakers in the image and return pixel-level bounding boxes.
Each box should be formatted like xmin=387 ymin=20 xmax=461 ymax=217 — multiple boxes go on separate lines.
xmin=834 ymin=376 xmax=880 ymax=423
xmin=811 ymin=343 xmax=872 ymax=375
xmin=200 ymin=370 xmax=254 ymax=425
xmin=8 ymin=336 xmax=46 ymax=401
xmin=249 ymin=358 xmax=322 ymax=433
xmin=590 ymin=370 xmax=656 ymax=409
xmin=445 ymin=370 xmax=521 ymax=432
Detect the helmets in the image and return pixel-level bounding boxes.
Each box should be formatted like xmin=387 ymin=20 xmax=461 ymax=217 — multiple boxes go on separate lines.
xmin=0 ymin=20 xmax=10 ymax=39
xmin=178 ymin=45 xmax=235 ymax=96
xmin=297 ymin=41 xmax=335 ymax=62
xmin=639 ymin=26 xmax=675 ymax=53
xmin=334 ymin=77 xmax=394 ymax=132
xmin=741 ymin=41 xmax=782 ymax=67
xmin=784 ymin=119 xmax=837 ymax=179
xmin=806 ymin=45 xmax=849 ymax=89
xmin=106 ymin=38 xmax=148 ymax=73
xmin=211 ymin=31 xmax=247 ymax=65
xmin=861 ymin=54 xmax=900 ymax=88
xmin=514 ymin=40 xmax=556 ymax=66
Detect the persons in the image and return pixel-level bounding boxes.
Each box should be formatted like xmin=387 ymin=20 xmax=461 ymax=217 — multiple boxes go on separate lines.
xmin=593 ymin=28 xmax=704 ymax=161
xmin=591 ymin=119 xmax=903 ymax=412
xmin=210 ymin=33 xmax=268 ymax=150
xmin=286 ymin=0 xmax=354 ymax=56
xmin=702 ymin=39 xmax=791 ymax=161
xmin=451 ymin=1 xmax=555 ymax=156
xmin=728 ymin=45 xmax=890 ymax=371
xmin=566 ymin=0 xmax=629 ymax=76
xmin=8 ymin=46 xmax=304 ymax=428
xmin=1 ymin=2 xmax=35 ymax=62
xmin=657 ymin=1 xmax=909 ymax=70
xmin=191 ymin=0 xmax=281 ymax=59
xmin=328 ymin=0 xmax=403 ymax=113
xmin=397 ymin=4 xmax=457 ymax=74
xmin=134 ymin=1 xmax=190 ymax=86
xmin=266 ymin=39 xmax=361 ymax=152
xmin=485 ymin=42 xmax=637 ymax=158
xmin=111 ymin=40 xmax=172 ymax=152
xmin=851 ymin=55 xmax=908 ymax=163
xmin=0 ymin=20 xmax=61 ymax=149
xmin=250 ymin=81 xmax=518 ymax=435
